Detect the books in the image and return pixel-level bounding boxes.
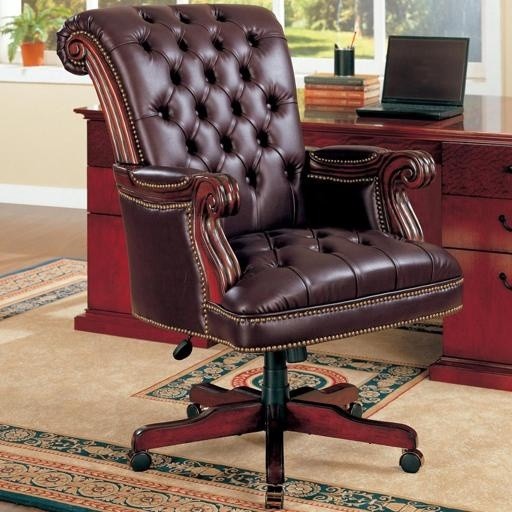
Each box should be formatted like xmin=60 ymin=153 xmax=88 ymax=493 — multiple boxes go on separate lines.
xmin=303 ymin=83 xmax=380 ymax=91
xmin=304 ymin=102 xmax=379 ymax=113
xmin=304 ymin=72 xmax=380 ymax=87
xmin=302 ymin=89 xmax=381 ymax=101
xmin=303 ymin=96 xmax=380 ymax=107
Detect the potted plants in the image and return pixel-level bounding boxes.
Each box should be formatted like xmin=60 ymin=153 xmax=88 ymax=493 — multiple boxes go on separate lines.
xmin=0 ymin=3 xmax=71 ymax=66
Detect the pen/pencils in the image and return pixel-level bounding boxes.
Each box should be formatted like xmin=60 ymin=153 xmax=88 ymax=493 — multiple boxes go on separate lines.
xmin=335 ymin=32 xmax=356 ymax=49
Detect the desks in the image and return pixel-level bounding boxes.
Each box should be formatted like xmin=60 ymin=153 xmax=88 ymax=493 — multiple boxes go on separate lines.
xmin=73 ymin=95 xmax=512 ymax=392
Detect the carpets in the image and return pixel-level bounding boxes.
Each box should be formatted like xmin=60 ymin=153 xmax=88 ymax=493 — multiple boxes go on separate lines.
xmin=0 ymin=255 xmax=512 ymax=512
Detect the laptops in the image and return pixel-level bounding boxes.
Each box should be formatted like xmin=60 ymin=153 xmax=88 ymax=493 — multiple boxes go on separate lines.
xmin=355 ymin=34 xmax=470 ymax=121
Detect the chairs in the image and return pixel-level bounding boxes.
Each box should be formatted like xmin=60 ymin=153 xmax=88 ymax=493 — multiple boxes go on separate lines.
xmin=57 ymin=4 xmax=465 ymax=510
